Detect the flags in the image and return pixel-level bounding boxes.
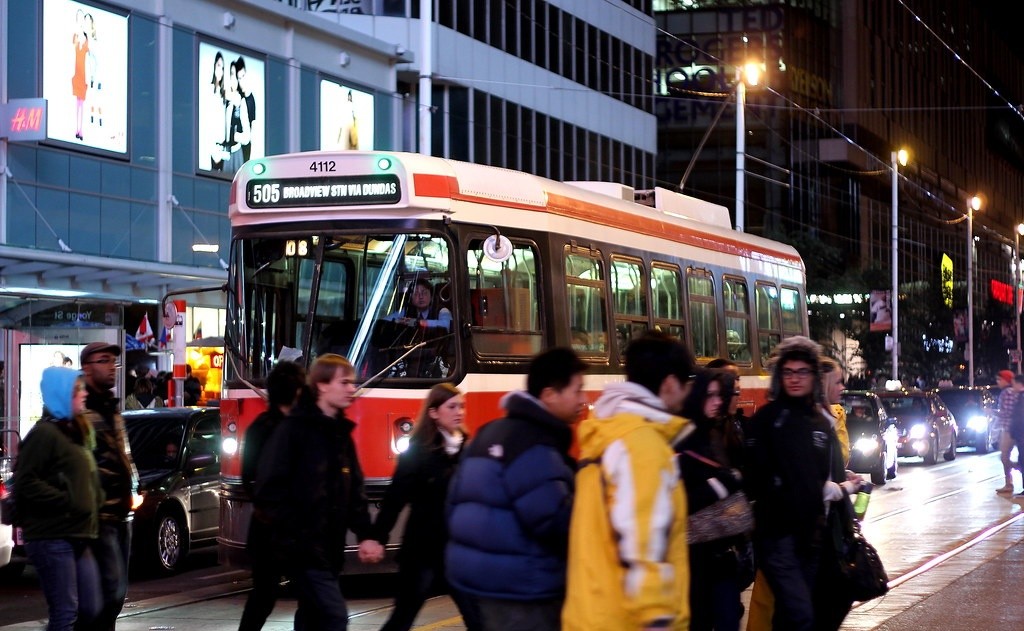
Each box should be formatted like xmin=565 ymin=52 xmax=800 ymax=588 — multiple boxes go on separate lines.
xmin=192 ymin=322 xmax=202 ymax=340
xmin=136 ymin=314 xmax=171 ymax=350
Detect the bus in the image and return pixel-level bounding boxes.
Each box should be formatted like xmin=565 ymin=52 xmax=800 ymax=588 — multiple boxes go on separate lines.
xmin=160 ymin=147 xmax=817 ymax=606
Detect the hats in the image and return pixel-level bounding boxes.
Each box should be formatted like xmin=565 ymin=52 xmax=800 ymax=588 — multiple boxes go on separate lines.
xmin=79 ymin=342 xmax=122 ymax=364
xmin=999 ymin=370 xmax=1016 ymax=384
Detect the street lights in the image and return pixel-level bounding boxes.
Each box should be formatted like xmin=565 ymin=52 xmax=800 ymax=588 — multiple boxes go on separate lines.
xmin=889 ymin=147 xmax=910 ymax=381
xmin=733 ymin=56 xmax=761 ymax=233
xmin=965 ymin=195 xmax=981 ymax=388
xmin=1013 ymin=222 xmax=1024 ymax=377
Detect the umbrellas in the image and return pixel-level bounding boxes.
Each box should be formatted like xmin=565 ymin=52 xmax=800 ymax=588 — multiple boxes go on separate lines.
xmin=32 ymin=316 xmax=145 ymax=352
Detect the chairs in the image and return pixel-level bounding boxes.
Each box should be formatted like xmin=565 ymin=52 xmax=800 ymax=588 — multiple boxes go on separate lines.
xmin=394 ymin=272 xmax=456 ymax=329
xmin=850 ymin=404 xmax=869 ymax=421
xmin=911 ymin=399 xmax=924 ymax=415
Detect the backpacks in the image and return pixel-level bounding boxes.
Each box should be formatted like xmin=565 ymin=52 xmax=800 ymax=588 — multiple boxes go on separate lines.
xmin=0 ymin=458 xmax=24 ymax=527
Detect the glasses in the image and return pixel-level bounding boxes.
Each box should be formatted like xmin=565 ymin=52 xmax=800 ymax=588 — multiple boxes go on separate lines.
xmin=85 ymin=358 xmax=117 ymax=368
xmin=781 ymin=370 xmax=813 ymax=380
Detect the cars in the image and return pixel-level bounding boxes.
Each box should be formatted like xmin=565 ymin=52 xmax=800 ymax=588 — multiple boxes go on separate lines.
xmin=921 ymin=379 xmax=1003 ymax=454
xmin=838 ymin=388 xmax=899 ymax=485
xmin=117 ymin=401 xmax=221 ymax=581
xmin=865 ymin=379 xmax=959 ymax=468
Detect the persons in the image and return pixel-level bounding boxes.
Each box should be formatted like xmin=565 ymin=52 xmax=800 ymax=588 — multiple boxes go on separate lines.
xmin=746 ymin=336 xmax=863 ymax=631
xmin=910 ymin=398 xmax=925 ymax=416
xmin=853 ymin=402 xmax=872 ymax=422
xmin=570 ymin=325 xmax=751 ymax=352
xmin=995 ymin=370 xmax=1024 ymax=495
xmin=11 ymin=341 xmax=133 ymax=631
xmin=236 ymin=352 xmax=382 ymax=631
xmin=72 ymin=9 xmax=102 ymax=140
xmin=669 ymin=358 xmax=749 ymax=631
xmin=124 ymin=363 xmax=201 ymax=410
xmin=53 ymin=350 xmax=73 ymax=369
xmin=204 ymin=52 xmax=258 ymax=174
xmin=381 ymin=277 xmax=452 ymax=332
xmin=560 ymin=329 xmax=696 ymax=631
xmin=847 ymin=368 xmax=953 ymax=390
xmin=449 ymin=348 xmax=588 ymax=631
xmin=361 ymin=381 xmax=475 ymax=631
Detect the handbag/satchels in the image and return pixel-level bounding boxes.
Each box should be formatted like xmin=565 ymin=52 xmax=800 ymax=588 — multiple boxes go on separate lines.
xmin=831 ymin=485 xmax=888 ymax=602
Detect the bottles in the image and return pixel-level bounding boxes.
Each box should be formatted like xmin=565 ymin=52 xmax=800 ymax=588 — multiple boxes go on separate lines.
xmin=853 ymin=482 xmax=873 ymax=520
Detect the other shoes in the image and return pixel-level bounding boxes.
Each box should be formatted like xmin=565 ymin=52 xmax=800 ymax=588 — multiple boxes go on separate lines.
xmin=996 ymin=486 xmax=1014 ymax=492
